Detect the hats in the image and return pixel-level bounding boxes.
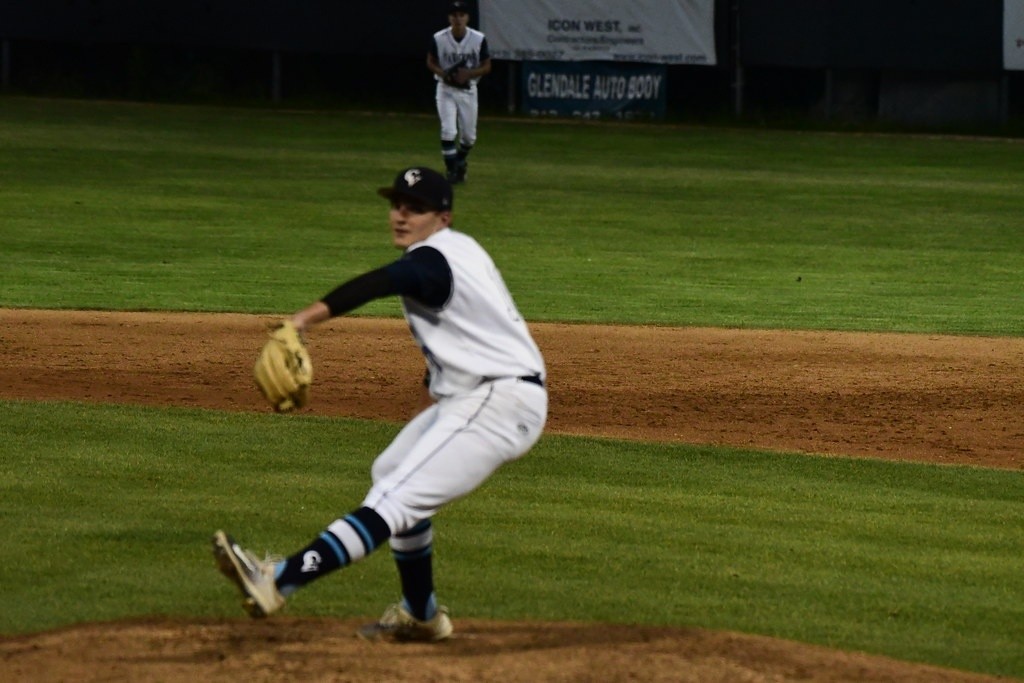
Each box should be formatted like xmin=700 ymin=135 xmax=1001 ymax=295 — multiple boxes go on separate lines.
xmin=447 ymin=2 xmax=472 ymax=18
xmin=377 ymin=165 xmax=454 ymax=214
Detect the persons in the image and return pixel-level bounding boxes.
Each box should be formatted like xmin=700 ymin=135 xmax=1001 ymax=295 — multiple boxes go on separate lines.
xmin=209 ymin=164 xmax=552 ymax=645
xmin=426 ymin=0 xmax=493 ymax=191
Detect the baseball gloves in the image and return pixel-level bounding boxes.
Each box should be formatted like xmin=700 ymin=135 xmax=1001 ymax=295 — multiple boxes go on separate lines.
xmin=253 ymin=320 xmax=312 ymax=412
xmin=443 ymin=60 xmax=472 ymax=90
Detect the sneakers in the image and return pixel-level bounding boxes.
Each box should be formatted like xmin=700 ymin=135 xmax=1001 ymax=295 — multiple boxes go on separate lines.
xmin=457 ymin=162 xmax=467 ymax=183
xmin=446 ymin=164 xmax=458 ymax=184
xmin=210 ymin=531 xmax=287 ymax=620
xmin=379 ymin=601 xmax=457 ymax=641
